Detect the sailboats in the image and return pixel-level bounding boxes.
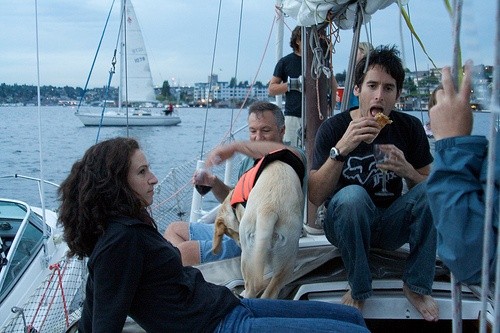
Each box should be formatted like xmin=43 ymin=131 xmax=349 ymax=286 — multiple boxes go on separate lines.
xmin=0 ymin=0 xmax=500 ymax=333
xmin=75 ymin=0 xmax=183 ymax=127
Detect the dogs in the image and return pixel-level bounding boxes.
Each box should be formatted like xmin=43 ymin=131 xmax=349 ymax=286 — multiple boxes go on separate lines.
xmin=213 ymin=148 xmax=305 ymax=299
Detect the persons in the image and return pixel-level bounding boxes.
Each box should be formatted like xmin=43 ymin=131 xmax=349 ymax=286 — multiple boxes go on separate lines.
xmin=58 ymin=136 xmax=372 ymax=333
xmin=162 ymin=103 xmax=309 ymax=266
xmin=310 ymin=45 xmax=500 ymax=322
xmin=268 ymin=25 xmax=374 ymax=144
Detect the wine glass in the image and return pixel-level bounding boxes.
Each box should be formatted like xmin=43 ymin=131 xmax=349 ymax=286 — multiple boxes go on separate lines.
xmin=194 ymin=160 xmax=213 ymax=216
xmin=373 ymin=144 xmax=394 ymax=196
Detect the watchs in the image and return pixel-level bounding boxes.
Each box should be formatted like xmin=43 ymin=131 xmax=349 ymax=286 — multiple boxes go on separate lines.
xmin=329 ymin=146 xmax=349 ymax=163
